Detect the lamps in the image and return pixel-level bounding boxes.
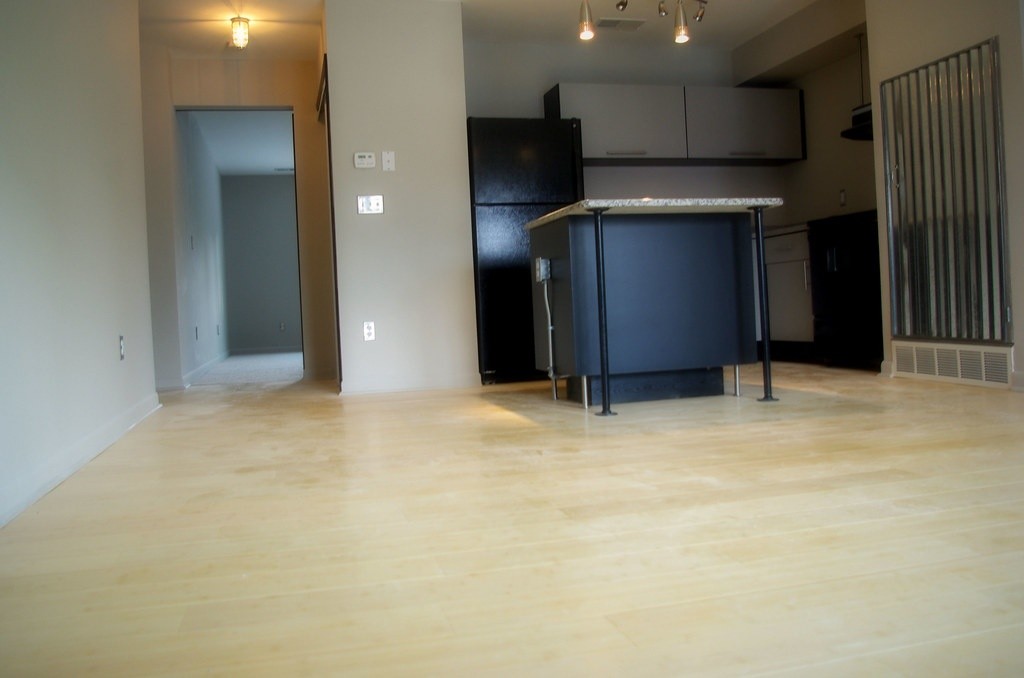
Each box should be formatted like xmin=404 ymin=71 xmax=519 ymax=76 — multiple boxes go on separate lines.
xmin=578 ymin=0 xmax=595 ymax=40
xmin=615 ymin=0 xmax=628 ymax=11
xmin=673 ymin=0 xmax=690 ymax=44
xmin=658 ymin=0 xmax=669 ymax=17
xmin=230 ymin=16 xmax=250 ymax=50
xmin=692 ymin=0 xmax=708 ymax=22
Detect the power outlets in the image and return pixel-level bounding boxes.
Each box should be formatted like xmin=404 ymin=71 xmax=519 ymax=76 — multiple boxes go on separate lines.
xmin=363 ymin=321 xmax=375 ymax=341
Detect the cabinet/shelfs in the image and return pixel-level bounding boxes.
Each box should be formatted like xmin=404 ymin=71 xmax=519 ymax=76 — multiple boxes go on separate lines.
xmin=685 ymin=84 xmax=808 ymax=166
xmin=544 ymin=83 xmax=687 ymax=168
xmin=525 ymin=198 xmax=783 ymax=416
xmin=761 ymin=230 xmax=814 ymax=344
